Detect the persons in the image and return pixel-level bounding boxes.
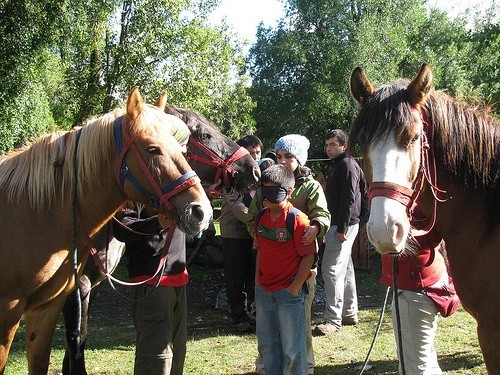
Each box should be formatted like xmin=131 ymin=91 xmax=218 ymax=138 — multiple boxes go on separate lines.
xmin=380 ymin=203 xmax=450 ymax=375
xmin=220 ymin=134 xmax=262 ymax=333
xmin=315 ymin=128 xmax=370 ymax=337
xmin=246 ymin=133 xmax=331 ymax=375
xmin=113 ymin=201 xmax=190 ymax=375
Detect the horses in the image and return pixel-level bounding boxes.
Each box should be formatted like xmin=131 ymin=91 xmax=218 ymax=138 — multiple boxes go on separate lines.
xmin=1 ymin=83 xmax=262 ymax=375
xmin=343 ymin=60 xmax=500 ymax=375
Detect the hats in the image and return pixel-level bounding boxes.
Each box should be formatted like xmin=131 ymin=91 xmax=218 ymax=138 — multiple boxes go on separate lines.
xmin=275 ymin=134 xmax=310 ymax=166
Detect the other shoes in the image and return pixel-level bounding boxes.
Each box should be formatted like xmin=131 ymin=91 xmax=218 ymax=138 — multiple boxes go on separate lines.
xmin=315 ymin=320 xmax=336 ymax=334
xmin=233 ymin=313 xmax=255 ymax=324
